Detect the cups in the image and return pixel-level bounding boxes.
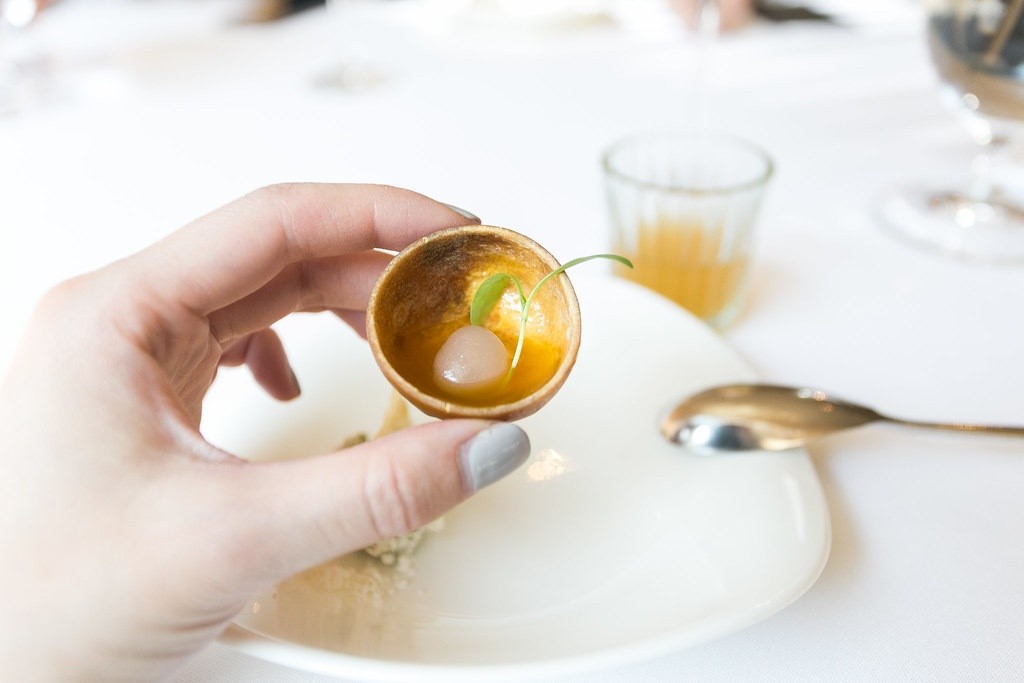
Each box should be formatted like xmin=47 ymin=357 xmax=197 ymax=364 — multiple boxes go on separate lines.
xmin=601 ymin=133 xmax=774 ymax=327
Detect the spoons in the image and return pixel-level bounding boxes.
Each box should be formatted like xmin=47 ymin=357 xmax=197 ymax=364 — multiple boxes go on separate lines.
xmin=661 ymin=380 xmax=1024 ymax=455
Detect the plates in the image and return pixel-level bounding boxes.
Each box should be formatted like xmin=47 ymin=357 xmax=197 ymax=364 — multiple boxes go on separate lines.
xmin=212 ymin=263 xmax=834 ymax=683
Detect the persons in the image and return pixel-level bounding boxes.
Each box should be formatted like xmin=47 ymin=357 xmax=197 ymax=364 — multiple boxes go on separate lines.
xmin=0 ymin=182 xmax=533 ymax=683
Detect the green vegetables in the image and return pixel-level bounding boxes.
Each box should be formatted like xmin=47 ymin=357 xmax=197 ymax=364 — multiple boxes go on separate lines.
xmin=469 ymin=253 xmax=634 ymax=369
